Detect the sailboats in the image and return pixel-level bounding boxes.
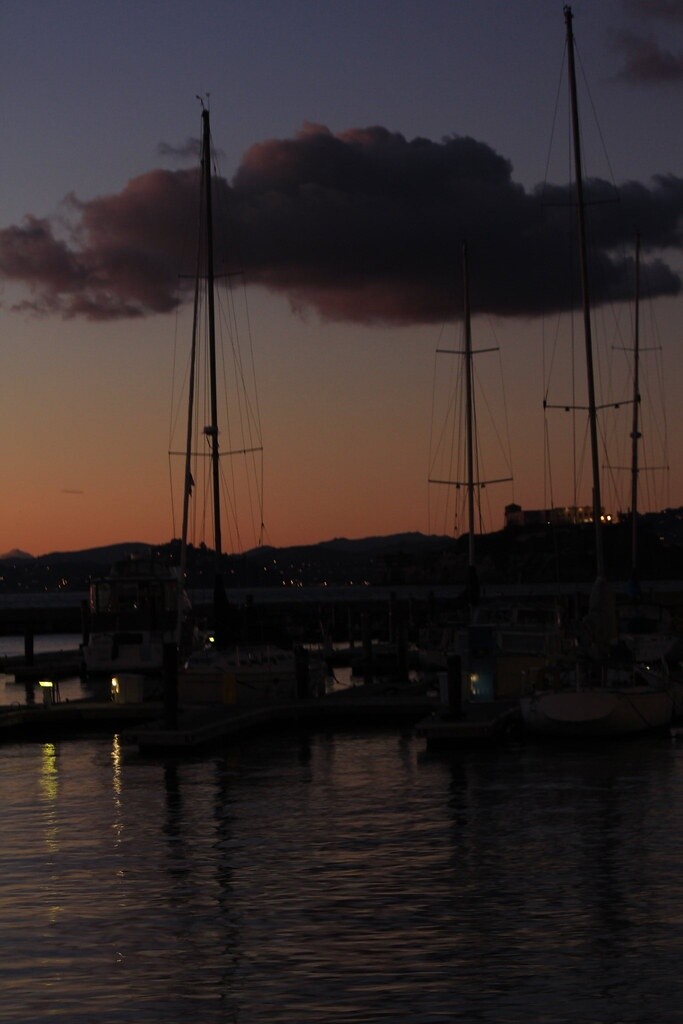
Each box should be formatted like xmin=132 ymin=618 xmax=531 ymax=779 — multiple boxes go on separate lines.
xmin=0 ymin=1 xmax=683 ymax=758
